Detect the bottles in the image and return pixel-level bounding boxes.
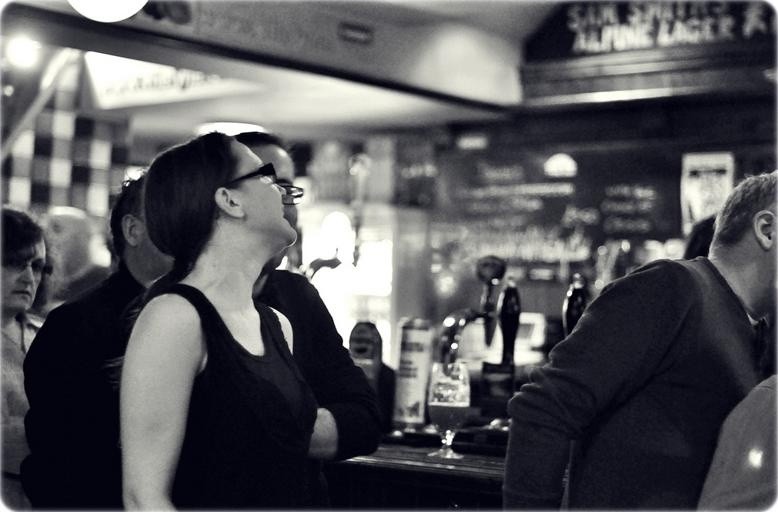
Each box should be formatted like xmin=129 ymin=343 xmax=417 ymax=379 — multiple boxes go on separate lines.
xmin=391 ymin=316 xmax=431 ymax=432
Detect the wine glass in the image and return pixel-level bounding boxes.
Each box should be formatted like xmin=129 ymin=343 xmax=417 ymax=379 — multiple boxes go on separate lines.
xmin=427 ymin=361 xmax=471 ymax=459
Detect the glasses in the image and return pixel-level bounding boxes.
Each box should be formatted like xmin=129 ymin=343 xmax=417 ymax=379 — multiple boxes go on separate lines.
xmin=281 ymin=184 xmax=306 ymax=205
xmin=219 ymin=163 xmax=278 ymax=188
